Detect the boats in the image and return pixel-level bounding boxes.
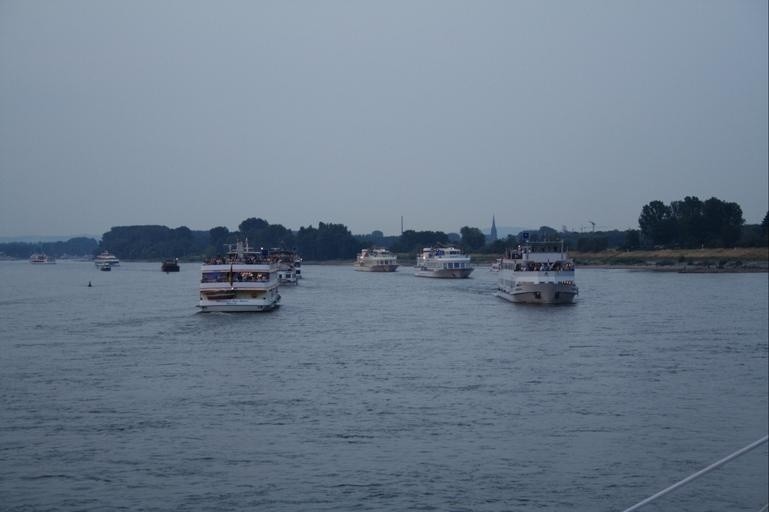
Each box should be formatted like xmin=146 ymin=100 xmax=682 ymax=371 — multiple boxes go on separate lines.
xmin=27 ymin=251 xmax=57 ymax=265
xmin=94 ymin=248 xmax=121 ymax=268
xmin=99 ymin=262 xmax=112 ymax=272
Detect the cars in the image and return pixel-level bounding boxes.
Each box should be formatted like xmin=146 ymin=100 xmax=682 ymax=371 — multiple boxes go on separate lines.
xmin=653 ymin=237 xmax=703 ymax=251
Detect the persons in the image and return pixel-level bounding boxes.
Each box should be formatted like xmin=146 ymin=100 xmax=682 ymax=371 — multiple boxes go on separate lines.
xmin=512 ymin=247 xmax=574 ymax=272
xmin=207 ymin=252 xmax=266 ymax=283
